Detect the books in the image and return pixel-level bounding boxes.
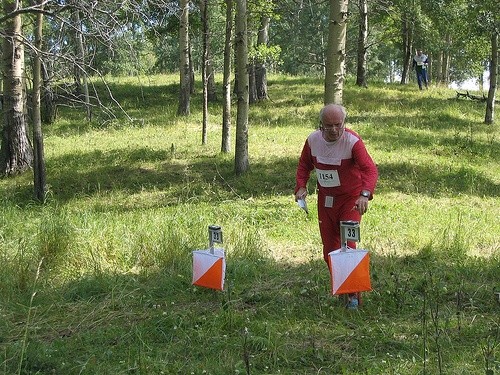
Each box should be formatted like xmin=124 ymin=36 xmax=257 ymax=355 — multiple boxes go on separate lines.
xmin=297 ymin=198 xmax=309 ymax=214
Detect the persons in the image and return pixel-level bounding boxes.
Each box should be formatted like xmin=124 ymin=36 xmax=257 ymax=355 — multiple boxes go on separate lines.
xmin=293 ymin=103 xmax=378 ymax=305
xmin=412 ymin=48 xmax=428 ymax=90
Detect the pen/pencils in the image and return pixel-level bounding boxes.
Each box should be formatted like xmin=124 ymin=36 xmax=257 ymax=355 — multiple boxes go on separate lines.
xmin=301 ymin=191 xmax=306 ymax=197
